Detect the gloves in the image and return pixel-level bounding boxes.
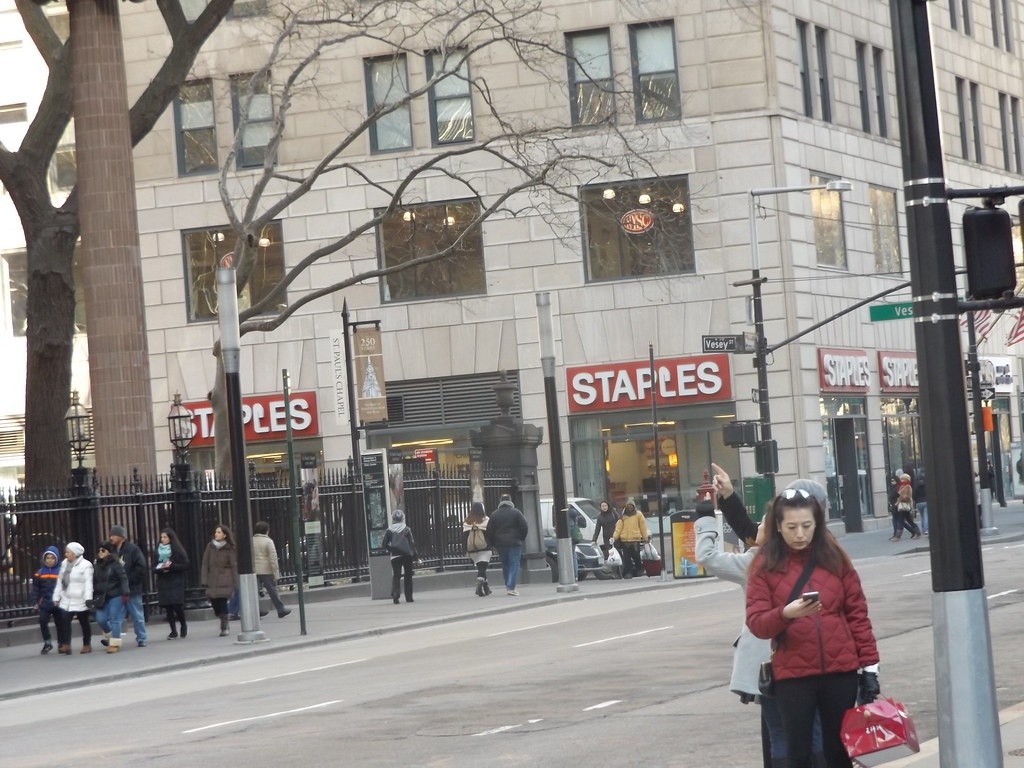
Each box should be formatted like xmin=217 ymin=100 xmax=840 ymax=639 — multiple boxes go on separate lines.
xmin=858 ymin=670 xmax=880 ymax=706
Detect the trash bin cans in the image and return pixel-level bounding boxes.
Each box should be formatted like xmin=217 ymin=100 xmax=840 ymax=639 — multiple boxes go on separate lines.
xmin=669 ymin=508 xmax=745 ymax=580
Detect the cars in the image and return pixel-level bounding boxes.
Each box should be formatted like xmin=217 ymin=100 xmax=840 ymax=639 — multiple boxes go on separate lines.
xmin=539 ymin=498 xmax=604 ymax=582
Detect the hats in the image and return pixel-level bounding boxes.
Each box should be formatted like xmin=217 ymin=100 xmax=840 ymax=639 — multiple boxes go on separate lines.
xmin=392 ymin=510 xmax=404 ymax=523
xmin=788 ymin=479 xmax=825 ymax=515
xmin=891 ymin=469 xmax=911 ymax=483
xmin=626 ymin=497 xmax=635 ymax=508
xmin=66 ymin=525 xmax=124 ymax=556
xmin=500 ymin=494 xmax=511 ymax=502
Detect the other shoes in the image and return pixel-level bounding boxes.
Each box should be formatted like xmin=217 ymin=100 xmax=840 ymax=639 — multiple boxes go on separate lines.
xmin=636 ymin=569 xmax=643 ymax=576
xmin=40 ymin=608 xmax=291 ymax=655
xmin=624 ymin=571 xmax=633 ymax=579
xmin=508 ymin=590 xmax=519 ymax=596
xmin=888 ymin=527 xmax=928 ymax=542
xmin=406 ymin=598 xmax=414 ymax=602
xmin=393 ymin=592 xmax=399 ymax=604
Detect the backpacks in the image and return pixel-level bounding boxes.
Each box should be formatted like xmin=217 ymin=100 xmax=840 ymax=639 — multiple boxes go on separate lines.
xmin=467 ymin=518 xmax=488 ymax=552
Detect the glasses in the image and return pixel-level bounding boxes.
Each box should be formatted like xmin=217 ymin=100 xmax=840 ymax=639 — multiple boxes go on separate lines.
xmin=781 ymin=488 xmax=812 ymax=500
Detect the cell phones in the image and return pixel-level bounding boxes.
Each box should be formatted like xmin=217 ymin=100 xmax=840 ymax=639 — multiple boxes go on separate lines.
xmin=802 ymin=591 xmax=819 ymax=605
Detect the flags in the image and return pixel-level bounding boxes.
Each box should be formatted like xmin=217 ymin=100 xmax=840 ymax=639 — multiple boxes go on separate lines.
xmin=1004 ymin=307 xmax=1024 ymax=346
xmin=961 ymin=309 xmax=991 ymax=340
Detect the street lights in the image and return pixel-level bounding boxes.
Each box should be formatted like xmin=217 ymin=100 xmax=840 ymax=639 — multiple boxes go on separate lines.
xmin=535 ymin=291 xmax=586 ymax=594
xmin=64 ymin=390 xmax=105 ymax=562
xmin=168 ymin=391 xmax=208 ymax=560
xmin=746 ymin=181 xmax=853 ymax=496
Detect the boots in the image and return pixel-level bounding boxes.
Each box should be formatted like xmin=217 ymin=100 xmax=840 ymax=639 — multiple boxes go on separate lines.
xmin=475 ymin=577 xmax=485 ymax=596
xmin=483 ymin=578 xmax=492 ymax=595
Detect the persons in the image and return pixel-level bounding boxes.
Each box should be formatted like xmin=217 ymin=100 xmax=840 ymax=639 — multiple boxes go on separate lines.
xmin=93 ymin=541 xmax=130 ymax=653
xmin=105 ymin=526 xmax=149 ymax=647
xmin=973 ymin=472 xmax=982 ymax=529
xmin=1017 ymin=452 xmax=1024 ymax=501
xmin=200 ymin=524 xmax=239 ymax=637
xmin=746 ymin=488 xmax=880 ymax=768
xmin=32 ymin=545 xmax=64 ymax=655
xmin=462 ymin=502 xmax=493 ymax=597
xmin=252 ymin=521 xmax=293 ymax=619
xmin=710 ymin=462 xmax=829 ymax=768
xmin=52 ymin=542 xmax=93 ymax=654
xmin=695 ymin=492 xmax=828 ymax=768
xmin=151 ymin=528 xmax=190 ymax=640
xmin=888 ymin=465 xmax=929 ymax=542
xmin=567 ymin=506 xmax=580 ymax=582
xmin=486 ymin=494 xmax=528 ymax=597
xmin=382 ymin=510 xmax=421 ymax=604
xmin=591 ymin=501 xmax=623 ymax=579
xmin=230 ymin=588 xmax=268 ymax=622
xmin=612 ymin=499 xmax=649 ymax=579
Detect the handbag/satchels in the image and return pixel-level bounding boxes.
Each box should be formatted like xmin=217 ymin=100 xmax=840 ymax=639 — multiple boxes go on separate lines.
xmin=897 ymin=501 xmax=911 ymax=512
xmin=93 ymin=592 xmax=107 ymax=608
xmin=571 ymin=525 xmax=582 ymax=544
xmin=840 ymin=693 xmax=920 ymax=768
xmin=642 ymin=543 xmax=661 ymax=560
xmin=390 ymin=531 xmax=413 ymax=555
xmin=607 ymin=543 xmax=623 ymax=566
xmin=887 ymin=506 xmax=895 ymax=513
xmin=758 ymin=660 xmax=774 ymax=696
